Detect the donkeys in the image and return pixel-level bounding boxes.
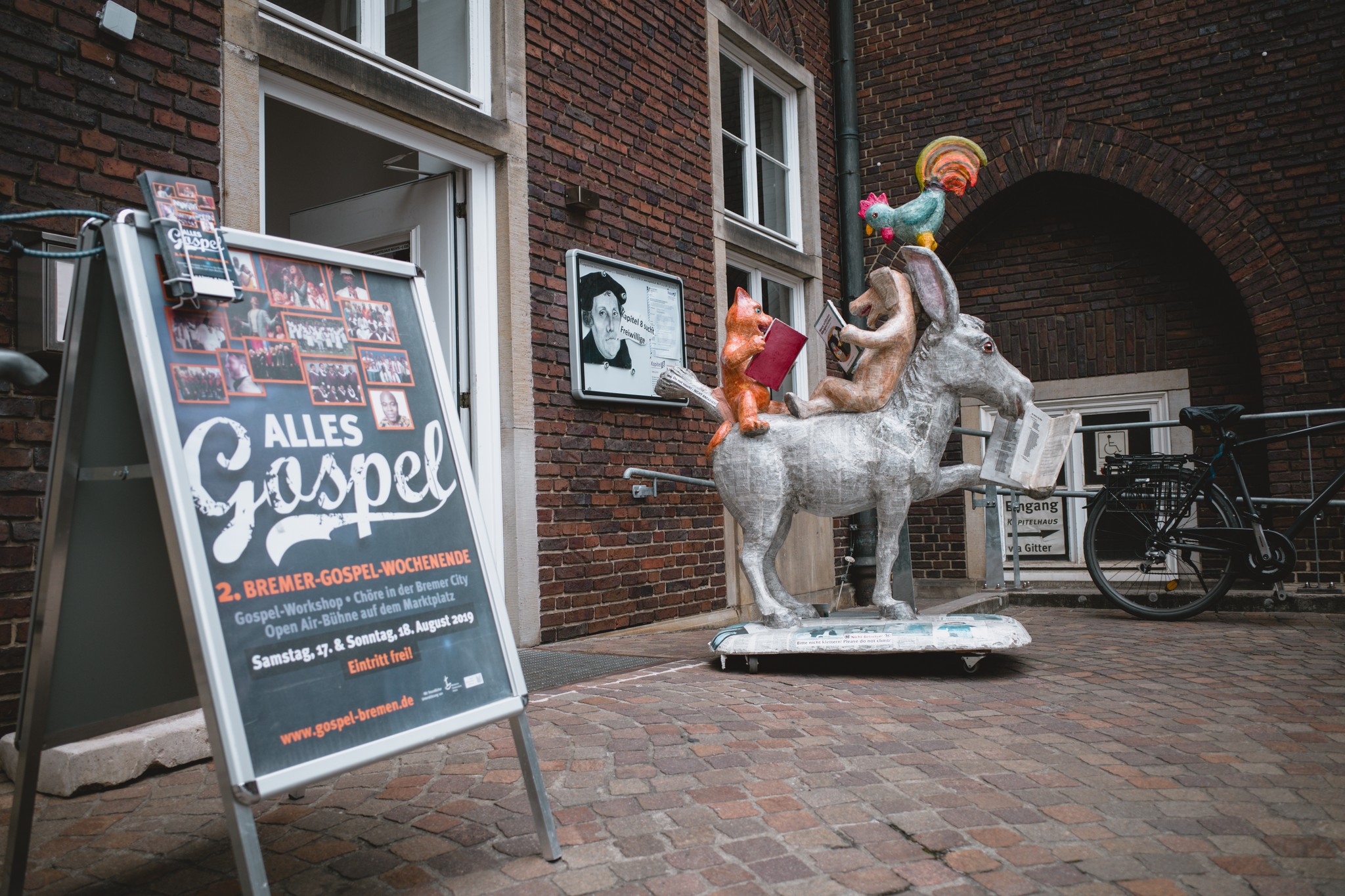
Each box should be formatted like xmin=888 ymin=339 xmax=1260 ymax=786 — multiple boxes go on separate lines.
xmin=654 ymin=245 xmax=1056 ymax=629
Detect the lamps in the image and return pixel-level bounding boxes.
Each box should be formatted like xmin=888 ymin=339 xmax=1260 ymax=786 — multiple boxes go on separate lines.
xmin=565 ymin=186 xmax=600 ymax=210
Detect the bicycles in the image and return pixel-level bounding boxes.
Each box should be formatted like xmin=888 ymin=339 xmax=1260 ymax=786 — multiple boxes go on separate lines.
xmin=1082 ymin=403 xmax=1345 ymax=622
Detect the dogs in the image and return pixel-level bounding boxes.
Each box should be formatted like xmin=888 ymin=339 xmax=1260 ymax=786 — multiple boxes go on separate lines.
xmin=784 ymin=266 xmax=917 ymax=419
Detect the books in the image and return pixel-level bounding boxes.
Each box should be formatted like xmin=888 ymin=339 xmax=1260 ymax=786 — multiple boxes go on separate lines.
xmin=814 ymin=299 xmax=866 ymax=376
xmin=744 ymin=317 xmax=809 ymax=392
xmin=978 ymin=400 xmax=1081 ymax=490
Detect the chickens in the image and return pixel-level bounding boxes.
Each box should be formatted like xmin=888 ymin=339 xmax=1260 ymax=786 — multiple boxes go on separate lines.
xmin=857 ymin=136 xmax=987 ymax=251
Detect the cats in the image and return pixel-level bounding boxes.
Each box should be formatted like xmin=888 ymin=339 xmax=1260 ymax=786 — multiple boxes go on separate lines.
xmin=705 ymin=287 xmax=790 ymax=478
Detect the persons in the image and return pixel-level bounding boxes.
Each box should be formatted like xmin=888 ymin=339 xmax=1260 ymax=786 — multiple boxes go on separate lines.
xmin=230 ymin=295 xmax=286 ymax=340
xmin=171 ymin=311 xmax=228 ymax=352
xmin=229 ymin=253 xmax=258 ymax=290
xmin=376 ymin=391 xmax=411 ymax=428
xmin=334 ymin=266 xmax=369 ymax=300
xmin=222 ymin=353 xmax=262 ymax=394
xmin=362 ymin=351 xmax=412 ymax=384
xmin=264 ymin=261 xmax=331 ymax=310
xmin=173 ymin=366 xmax=227 ymax=402
xmin=342 ymin=300 xmax=398 ymax=342
xmin=248 ymin=342 xmax=303 ymax=381
xmin=284 ymin=314 xmax=352 ymax=356
xmin=153 ymin=181 xmax=218 ymax=234
xmin=307 ymin=362 xmax=363 ymax=404
